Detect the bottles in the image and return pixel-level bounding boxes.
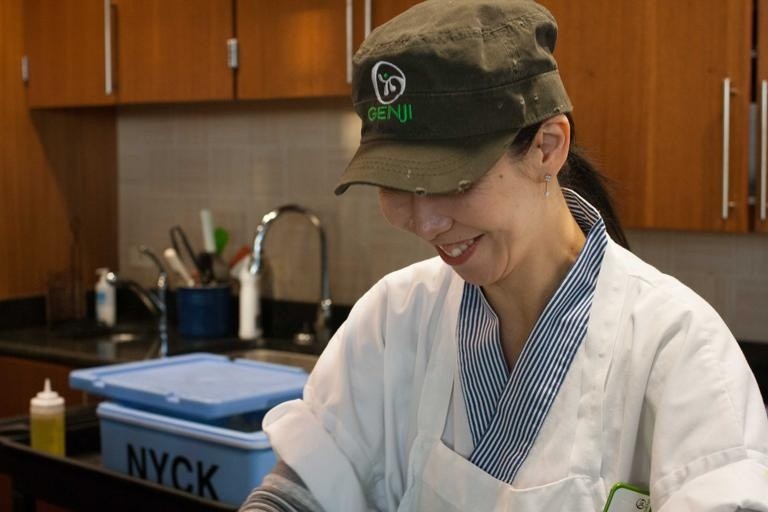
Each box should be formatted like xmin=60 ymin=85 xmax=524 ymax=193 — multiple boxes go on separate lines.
xmin=94 ymin=267 xmax=118 ymax=328
xmin=241 ymin=250 xmax=263 ymax=341
xmin=28 ymin=378 xmax=66 ymax=461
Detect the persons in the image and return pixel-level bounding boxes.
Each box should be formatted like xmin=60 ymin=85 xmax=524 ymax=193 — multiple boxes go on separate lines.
xmin=237 ymin=0 xmax=768 ymax=510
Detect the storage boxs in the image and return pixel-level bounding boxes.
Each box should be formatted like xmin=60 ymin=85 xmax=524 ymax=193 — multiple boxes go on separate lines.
xmin=69 ymin=353 xmax=309 ymax=509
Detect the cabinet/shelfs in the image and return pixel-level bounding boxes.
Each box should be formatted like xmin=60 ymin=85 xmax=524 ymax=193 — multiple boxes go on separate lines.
xmin=20 ymin=1 xmax=234 ymax=111
xmin=534 ymin=2 xmax=767 ymax=236
xmin=234 ymin=0 xmax=424 ymax=103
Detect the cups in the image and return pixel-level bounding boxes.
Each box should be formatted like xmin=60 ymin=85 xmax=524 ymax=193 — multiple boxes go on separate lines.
xmin=172 ymin=285 xmax=241 ymax=337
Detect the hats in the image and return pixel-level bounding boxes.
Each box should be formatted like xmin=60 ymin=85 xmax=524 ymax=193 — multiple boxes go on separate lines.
xmin=333 ymin=1 xmax=574 ymax=196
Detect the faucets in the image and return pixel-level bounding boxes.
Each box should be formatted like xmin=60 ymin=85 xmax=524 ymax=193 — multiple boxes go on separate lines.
xmin=247 ymin=203 xmax=333 ymax=355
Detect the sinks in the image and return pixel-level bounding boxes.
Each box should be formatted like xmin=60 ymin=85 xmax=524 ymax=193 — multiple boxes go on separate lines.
xmin=226 ymin=342 xmax=322 ymax=374
